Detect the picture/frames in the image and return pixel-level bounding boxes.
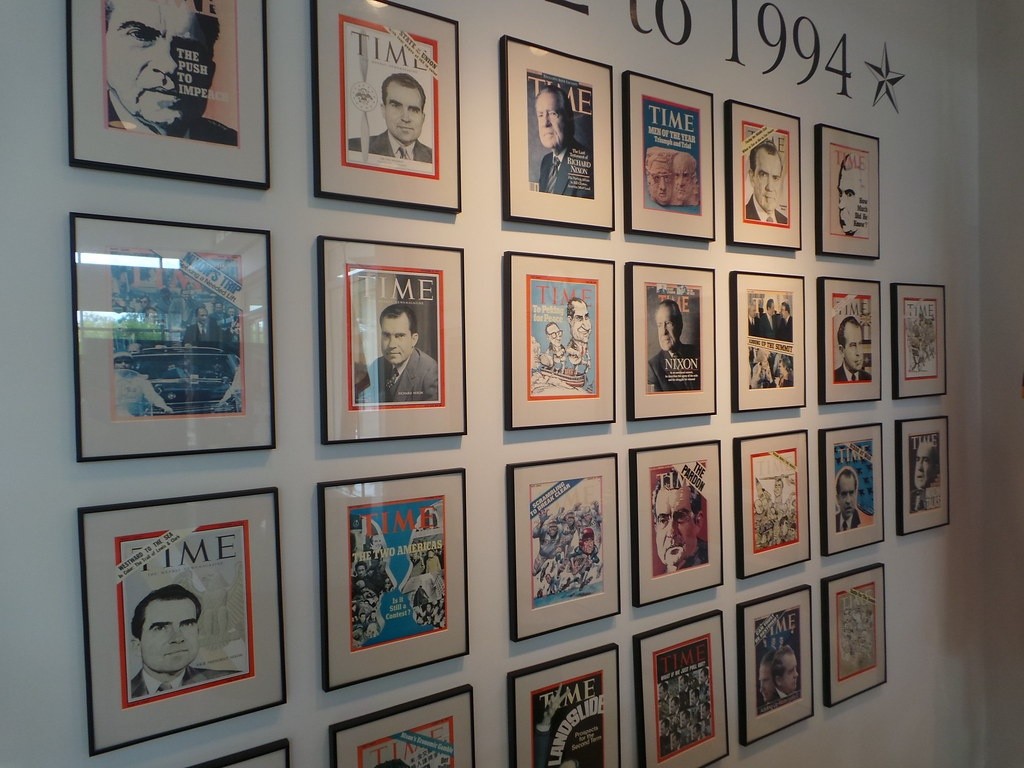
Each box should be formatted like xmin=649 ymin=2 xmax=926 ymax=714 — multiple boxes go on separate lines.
xmin=76 ymin=486 xmax=289 ymax=756
xmin=63 ymin=0 xmax=271 ymax=187
xmin=729 ymin=270 xmax=806 ymax=415
xmin=814 ymin=123 xmax=881 ymax=261
xmin=500 ymin=33 xmax=614 ymax=233
xmin=316 ymin=466 xmax=471 ymax=693
xmin=722 ymin=98 xmax=803 ymax=252
xmin=733 ymin=429 xmax=812 ymax=582
xmin=816 ymin=275 xmax=882 ymax=406
xmin=624 ymin=261 xmax=717 ymax=422
xmin=506 ymin=453 xmax=621 ymax=643
xmin=622 ymin=69 xmax=715 ymax=241
xmin=893 ymin=415 xmax=951 ymax=537
xmin=314 ymin=235 xmax=468 ymax=444
xmin=310 ymin=0 xmax=462 ymax=213
xmin=627 ymin=440 xmax=724 ymax=608
xmin=503 ymin=249 xmax=617 ymax=430
xmin=67 ymin=211 xmax=276 ymax=461
xmin=817 ymin=422 xmax=885 ymax=558
xmin=182 ymin=559 xmax=889 ymax=768
xmin=889 ymin=281 xmax=948 ymax=401
